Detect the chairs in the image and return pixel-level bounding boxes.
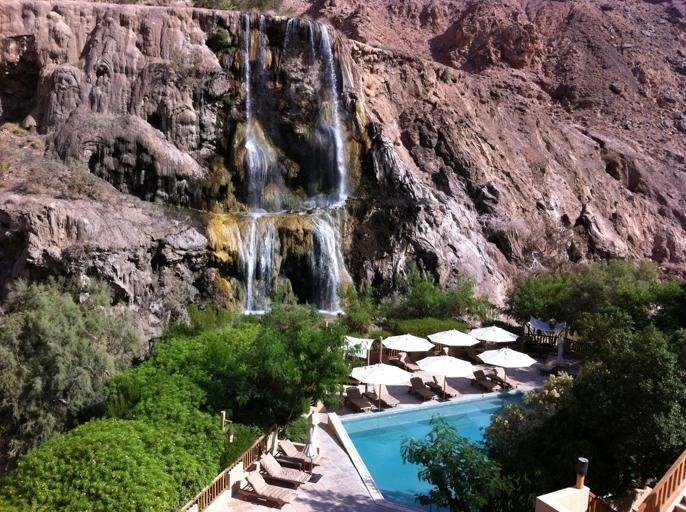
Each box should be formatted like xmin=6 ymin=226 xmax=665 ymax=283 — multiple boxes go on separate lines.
xmin=238 ymin=438 xmax=326 ymax=507
xmin=344 ymin=340 xmax=521 ymax=412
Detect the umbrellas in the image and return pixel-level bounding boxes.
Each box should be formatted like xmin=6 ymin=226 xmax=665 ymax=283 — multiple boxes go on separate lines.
xmin=418 ymin=354 xmax=476 ymax=400
xmin=468 ymin=325 xmax=519 ymax=344
xmin=382 ymin=332 xmax=436 ymax=355
xmin=348 ymin=362 xmax=413 ymax=413
xmin=427 ymin=327 xmax=480 ymax=349
xmin=477 ymin=348 xmax=538 ymax=392
